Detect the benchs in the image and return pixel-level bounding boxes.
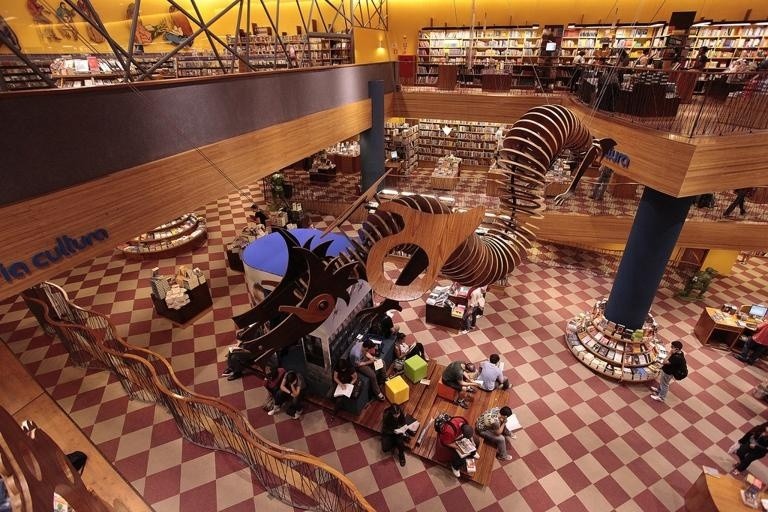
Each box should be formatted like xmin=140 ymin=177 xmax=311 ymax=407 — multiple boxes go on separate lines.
xmin=337 ymin=322 xmax=398 ymax=417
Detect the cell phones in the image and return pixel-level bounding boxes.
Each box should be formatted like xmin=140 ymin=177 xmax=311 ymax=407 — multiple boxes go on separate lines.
xmin=676 ymin=348 xmax=679 ymax=351
xmin=505 ymin=416 xmax=508 ymax=421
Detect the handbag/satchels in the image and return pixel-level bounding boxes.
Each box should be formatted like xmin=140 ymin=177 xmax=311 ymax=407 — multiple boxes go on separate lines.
xmin=672 ymin=354 xmax=688 ymax=380
xmin=393 ymin=358 xmax=404 ymax=371
xmin=729 ymin=443 xmax=740 ymax=454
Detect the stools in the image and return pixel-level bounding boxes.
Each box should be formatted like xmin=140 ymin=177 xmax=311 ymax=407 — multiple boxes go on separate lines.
xmin=384 ymin=354 xmax=459 ymax=464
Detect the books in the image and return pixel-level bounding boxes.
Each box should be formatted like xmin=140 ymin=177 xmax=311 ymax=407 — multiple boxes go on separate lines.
xmin=544 ymin=158 xmax=571 ymax=181
xmin=466 ymin=458 xmax=476 ymax=473
xmin=504 ymin=413 xmax=521 ymax=432
xmin=118 ymin=211 xmax=207 ymax=254
xmin=472 ymin=380 xmax=484 ymax=386
xmin=384 ymin=118 xmax=498 ymax=176
xmin=416 ymin=27 xmax=767 ymax=88
xmin=226 ymin=34 xmax=351 ymax=74
xmin=394 ymin=423 xmax=408 ymax=437
xmin=150 ymin=266 xmax=205 ymax=308
xmin=455 ymin=438 xmax=476 ymax=459
xmin=566 ymin=298 xmax=666 ymax=380
xmin=426 ymin=279 xmax=467 ymax=318
xmin=496 ymin=361 xmax=504 ymax=371
xmin=1 ymin=51 xmax=234 ymax=90
xmin=739 ymin=473 xmax=768 ymax=512
xmin=226 ymin=202 xmax=303 ymax=254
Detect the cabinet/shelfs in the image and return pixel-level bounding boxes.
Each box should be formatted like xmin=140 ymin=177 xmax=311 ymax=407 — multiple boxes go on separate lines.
xmin=228 ymin=32 xmax=353 ymax=74
xmin=565 ymin=297 xmax=669 ymax=389
xmin=383 ymin=118 xmax=506 ymax=178
xmin=116 ymin=210 xmax=209 ymax=262
xmin=0 ymin=55 xmax=228 ymax=92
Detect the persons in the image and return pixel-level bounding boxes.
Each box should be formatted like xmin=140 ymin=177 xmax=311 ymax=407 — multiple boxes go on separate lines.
xmin=650 ymin=340 xmax=683 ymax=401
xmin=722 ymin=189 xmax=751 ymax=216
xmin=477 ymin=353 xmax=511 ymax=392
xmin=734 ymin=318 xmax=768 ymax=364
xmin=462 ymin=287 xmax=485 ymax=334
xmin=381 ymin=403 xmax=408 ymax=466
xmin=302 ymin=213 xmax=314 ymax=230
xmin=221 ymin=302 xmax=429 ymax=421
xmin=476 ymin=405 xmax=518 ymax=460
xmin=590 ymin=164 xmax=614 ymax=200
xmin=442 ymin=360 xmax=481 ymax=408
xmin=733 ymin=421 xmax=767 ymax=471
xmin=476 ymin=285 xmax=489 ymax=317
xmin=436 ymin=416 xmax=480 ymax=477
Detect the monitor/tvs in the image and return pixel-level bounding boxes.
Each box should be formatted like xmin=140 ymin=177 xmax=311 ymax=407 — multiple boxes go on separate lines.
xmin=749 ymin=304 xmax=768 ymax=317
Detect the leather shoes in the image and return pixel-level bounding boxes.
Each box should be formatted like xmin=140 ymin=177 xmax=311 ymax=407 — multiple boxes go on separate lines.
xmin=228 ymin=372 xmax=243 ymax=381
xmin=223 ymin=368 xmax=231 ymax=374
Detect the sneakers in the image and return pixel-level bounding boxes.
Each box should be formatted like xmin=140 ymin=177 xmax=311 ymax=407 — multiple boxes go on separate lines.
xmin=650 ymin=395 xmax=663 ymax=402
xmin=466 ymin=386 xmax=476 ymax=392
xmin=400 ymin=454 xmax=405 ymax=466
xmin=268 ymin=406 xmax=280 ymax=416
xmin=651 ymin=387 xmax=658 ymax=392
xmin=476 ymin=315 xmax=482 ymax=318
xmin=295 ymin=410 xmax=303 ymax=419
xmin=458 ymin=325 xmax=475 ymax=334
xmin=474 ymin=453 xmax=480 ymax=459
xmin=378 ymin=393 xmax=385 ymax=401
xmin=498 ymin=456 xmax=512 ymax=461
xmin=458 ymin=398 xmax=468 ymax=408
xmin=452 ymin=465 xmax=461 ymax=477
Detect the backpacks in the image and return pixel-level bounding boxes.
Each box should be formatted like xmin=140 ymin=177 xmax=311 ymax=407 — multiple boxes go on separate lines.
xmin=435 ymin=413 xmax=467 ymax=433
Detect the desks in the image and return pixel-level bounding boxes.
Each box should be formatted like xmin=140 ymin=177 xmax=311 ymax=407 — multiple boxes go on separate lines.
xmin=693 ymin=305 xmax=768 ymax=351
